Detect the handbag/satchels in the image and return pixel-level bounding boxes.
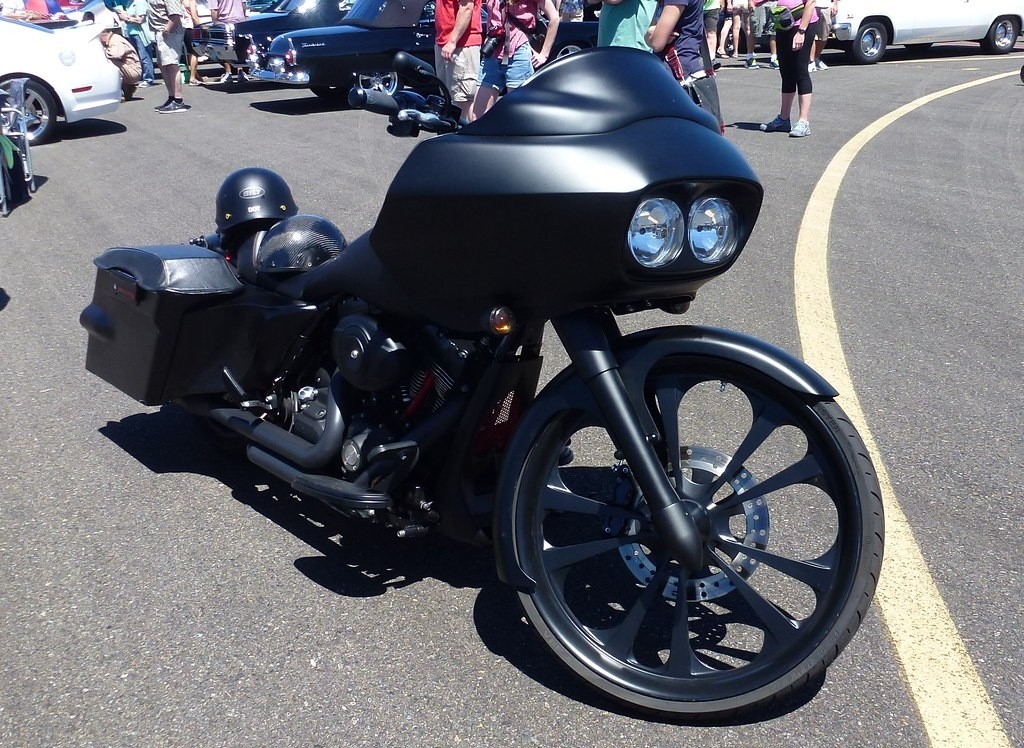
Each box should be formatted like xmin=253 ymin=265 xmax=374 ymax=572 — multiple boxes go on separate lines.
xmin=499 ymin=0 xmax=558 ymax=59
xmin=681 ymin=76 xmax=724 ymax=135
xmin=770 ymin=5 xmax=795 ymax=32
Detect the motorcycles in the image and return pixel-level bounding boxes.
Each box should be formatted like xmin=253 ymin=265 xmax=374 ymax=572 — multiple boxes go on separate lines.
xmin=81 ymin=45 xmax=885 ymax=725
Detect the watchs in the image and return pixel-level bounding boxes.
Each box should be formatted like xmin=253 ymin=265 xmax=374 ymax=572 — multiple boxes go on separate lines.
xmin=164 ymin=29 xmax=169 ymax=33
xmin=799 ymin=28 xmax=806 ymax=35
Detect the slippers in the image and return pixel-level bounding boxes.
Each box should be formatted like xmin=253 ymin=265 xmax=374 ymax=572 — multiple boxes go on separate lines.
xmin=716 ymin=51 xmax=730 ymax=59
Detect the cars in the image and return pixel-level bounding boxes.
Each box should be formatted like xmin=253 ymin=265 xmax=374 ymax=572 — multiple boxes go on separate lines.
xmin=248 ymin=0 xmax=273 ymax=13
xmin=828 ymin=0 xmax=1024 ymax=65
xmin=62 ymin=0 xmax=259 ymax=65
xmin=251 ymin=0 xmax=599 ymax=100
xmin=0 ymin=16 xmax=122 ymax=145
xmin=191 ymin=0 xmax=386 ymax=83
xmin=56 ymin=0 xmax=85 ymax=12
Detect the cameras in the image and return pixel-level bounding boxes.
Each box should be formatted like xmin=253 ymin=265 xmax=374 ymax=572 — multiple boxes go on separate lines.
xmin=480 ymin=35 xmax=501 ymax=58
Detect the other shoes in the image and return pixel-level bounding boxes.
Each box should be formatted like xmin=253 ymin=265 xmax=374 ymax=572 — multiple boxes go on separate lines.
xmin=120 ymin=89 xmax=126 ymax=100
xmin=711 ymin=59 xmax=722 ymax=70
xmin=753 ymin=53 xmax=756 ymax=56
xmin=189 ymin=76 xmax=208 ymax=86
xmin=139 ymin=80 xmax=151 ymax=88
xmin=732 ymin=52 xmax=739 ymax=59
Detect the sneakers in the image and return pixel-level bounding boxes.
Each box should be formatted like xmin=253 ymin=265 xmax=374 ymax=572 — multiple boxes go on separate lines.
xmin=153 ymin=100 xmax=187 ymax=114
xmin=760 ymin=115 xmax=791 ymax=133
xmin=808 ymin=61 xmax=828 ymax=72
xmin=744 ymin=58 xmax=760 ymax=70
xmin=789 ymin=120 xmax=811 ymax=137
xmin=237 ymin=70 xmax=249 ymax=83
xmin=769 ymin=59 xmax=779 ymax=70
xmin=220 ymin=73 xmax=233 ymax=83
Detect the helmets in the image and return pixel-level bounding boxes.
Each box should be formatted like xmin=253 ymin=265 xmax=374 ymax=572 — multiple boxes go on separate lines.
xmin=254 ymin=215 xmax=347 ymax=278
xmin=215 ymin=168 xmax=299 ymax=233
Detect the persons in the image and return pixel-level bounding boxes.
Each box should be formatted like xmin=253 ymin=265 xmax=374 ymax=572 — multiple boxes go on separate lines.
xmin=0 ymin=0 xmax=250 ymax=113
xmin=434 ymin=0 xmax=838 ymax=137
